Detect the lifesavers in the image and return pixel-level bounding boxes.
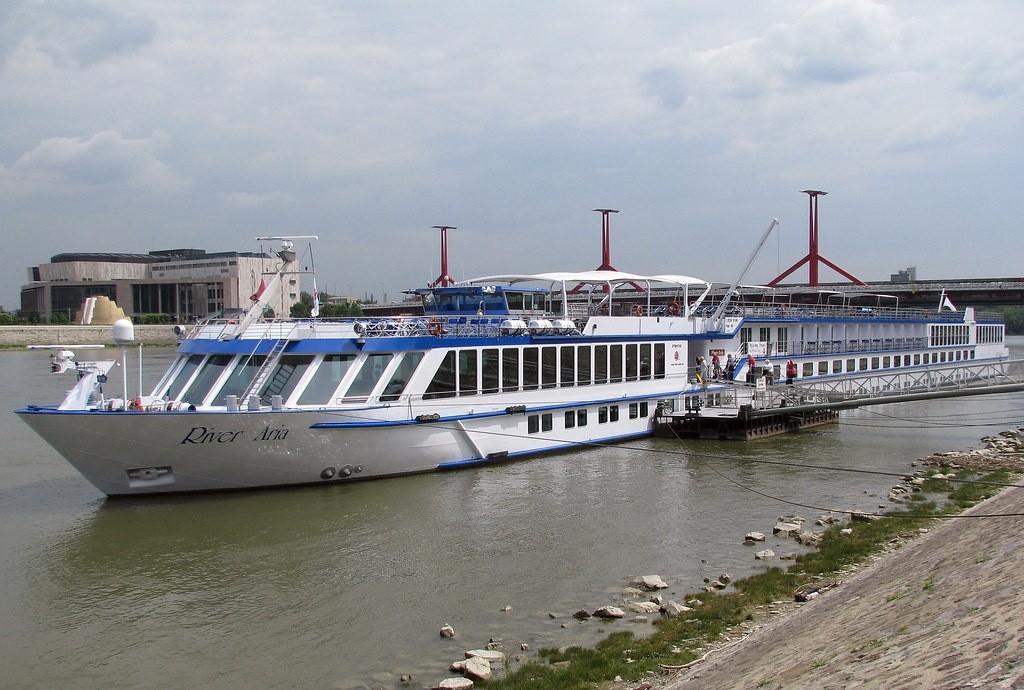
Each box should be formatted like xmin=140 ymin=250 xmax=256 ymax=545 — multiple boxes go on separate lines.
xmin=130 ymin=402 xmax=143 ymax=411
xmin=637 ymin=306 xmax=643 ymax=316
xmin=429 ymin=318 xmax=442 ymax=335
xmin=668 ymin=303 xmax=679 ymax=314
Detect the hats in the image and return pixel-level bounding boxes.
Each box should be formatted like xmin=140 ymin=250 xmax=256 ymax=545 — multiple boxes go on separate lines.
xmin=711 ymin=351 xmax=714 ymax=355
xmin=700 ymin=357 xmax=704 ymax=360
xmin=765 ymin=360 xmax=770 ymax=363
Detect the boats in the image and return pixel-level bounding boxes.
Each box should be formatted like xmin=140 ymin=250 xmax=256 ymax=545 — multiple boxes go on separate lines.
xmin=14 ymin=219 xmax=1010 ymax=499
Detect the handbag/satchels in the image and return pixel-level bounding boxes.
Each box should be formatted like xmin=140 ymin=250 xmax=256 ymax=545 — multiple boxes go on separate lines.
xmin=726 ymin=360 xmax=733 ymax=371
xmin=717 ymin=364 xmax=720 ymax=368
xmin=763 ymin=369 xmax=768 ymax=376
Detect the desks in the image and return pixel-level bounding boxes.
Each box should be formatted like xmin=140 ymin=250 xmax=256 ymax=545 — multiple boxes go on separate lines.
xmin=366 ymin=325 xmax=397 ymax=336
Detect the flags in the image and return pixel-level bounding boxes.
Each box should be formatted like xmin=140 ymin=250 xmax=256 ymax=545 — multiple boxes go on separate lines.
xmin=311 ymin=278 xmax=319 ymax=317
xmin=944 ymin=297 xmax=956 ymax=312
xmin=249 ymin=279 xmax=267 ymax=301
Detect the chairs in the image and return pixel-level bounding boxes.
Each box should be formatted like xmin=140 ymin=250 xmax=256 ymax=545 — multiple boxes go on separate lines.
xmin=435 ymin=318 xmax=520 ymax=337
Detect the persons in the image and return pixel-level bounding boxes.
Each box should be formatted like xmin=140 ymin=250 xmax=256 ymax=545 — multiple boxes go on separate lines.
xmin=696 ymin=350 xmax=796 ymax=384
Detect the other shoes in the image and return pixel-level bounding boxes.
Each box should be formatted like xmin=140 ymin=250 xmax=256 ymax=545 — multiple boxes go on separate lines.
xmin=751 ymin=385 xmax=755 ymax=387
xmin=744 ymin=385 xmax=749 ymax=386
